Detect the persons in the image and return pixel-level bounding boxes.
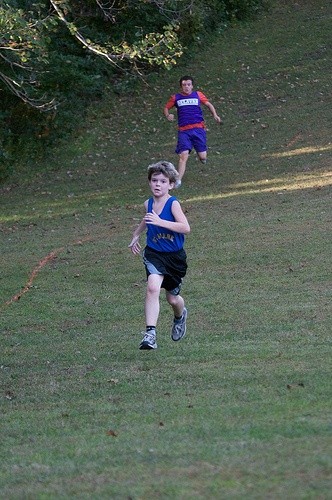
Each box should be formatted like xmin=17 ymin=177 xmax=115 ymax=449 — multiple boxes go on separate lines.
xmin=128 ymin=161 xmax=190 ymax=350
xmin=164 ymin=76 xmax=221 ymax=190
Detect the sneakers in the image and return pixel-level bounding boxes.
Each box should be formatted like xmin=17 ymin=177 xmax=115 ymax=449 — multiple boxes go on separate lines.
xmin=139 ymin=331 xmax=157 ymax=350
xmin=200 ymin=159 xmax=206 ymax=164
xmin=173 ymin=180 xmax=181 ymax=189
xmin=171 ymin=308 xmax=187 ymax=341
